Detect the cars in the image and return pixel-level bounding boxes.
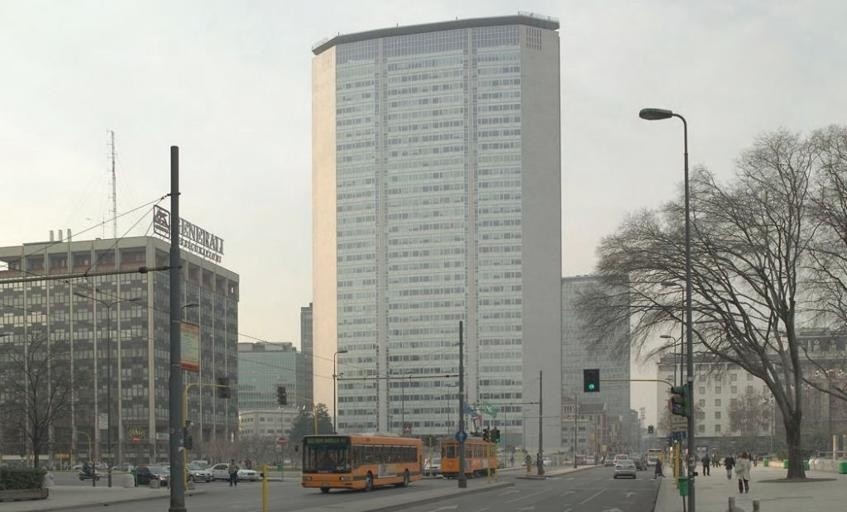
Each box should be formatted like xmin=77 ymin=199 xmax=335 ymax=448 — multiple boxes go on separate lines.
xmin=604 ymin=454 xmax=648 ymax=478
xmin=186 ymin=458 xmax=259 ymax=482
xmin=73 ymin=463 xmax=132 ymax=471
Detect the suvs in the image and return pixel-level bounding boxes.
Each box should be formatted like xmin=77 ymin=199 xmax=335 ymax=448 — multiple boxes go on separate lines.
xmin=134 ymin=466 xmax=169 ymax=485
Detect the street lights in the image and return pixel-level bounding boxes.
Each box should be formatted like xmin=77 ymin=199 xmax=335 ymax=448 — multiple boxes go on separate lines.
xmin=639 ymin=109 xmax=696 ymax=510
xmin=180 ymin=301 xmax=200 ymax=452
xmin=660 ymin=335 xmax=678 ymax=444
xmin=332 ymin=351 xmax=347 ymax=434
xmin=76 ymin=292 xmax=141 ymax=467
xmin=661 ymin=281 xmax=685 ymax=424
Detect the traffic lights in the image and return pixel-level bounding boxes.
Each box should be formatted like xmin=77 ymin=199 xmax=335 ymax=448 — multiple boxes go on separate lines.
xmin=278 ymin=388 xmax=287 ymax=404
xmin=671 ymin=386 xmax=684 ymax=417
xmin=583 ymin=369 xmax=600 ymax=392
xmin=483 ymin=429 xmax=500 ymax=443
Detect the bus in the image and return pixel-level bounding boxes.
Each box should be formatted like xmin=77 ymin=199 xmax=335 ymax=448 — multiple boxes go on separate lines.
xmin=441 ymin=439 xmax=496 ymax=479
xmin=648 ymin=449 xmax=661 ymax=466
xmin=302 ymin=434 xmax=425 ymax=492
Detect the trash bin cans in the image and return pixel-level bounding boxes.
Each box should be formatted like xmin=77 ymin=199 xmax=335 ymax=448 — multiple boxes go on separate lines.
xmin=784 ymin=457 xmax=788 ymax=469
xmin=763 ymin=456 xmax=768 ymax=466
xmin=803 ymin=460 xmax=810 ymax=471
xmin=838 ymin=461 xmax=847 ymax=474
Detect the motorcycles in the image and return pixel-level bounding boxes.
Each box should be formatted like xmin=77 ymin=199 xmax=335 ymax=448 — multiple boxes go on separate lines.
xmin=79 ymin=470 xmax=98 ymax=480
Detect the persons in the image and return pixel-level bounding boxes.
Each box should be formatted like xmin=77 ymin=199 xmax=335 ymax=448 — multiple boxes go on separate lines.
xmin=701 ymin=448 xmax=721 ymax=476
xmin=82 ymin=462 xmax=91 ymax=475
xmin=724 ymin=453 xmax=736 ymax=480
xmin=228 ymin=459 xmax=239 ymax=487
xmin=735 ymin=451 xmax=752 ymax=494
xmin=524 ymin=455 xmax=532 ymax=472
xmin=654 ymin=458 xmax=666 ymax=479
xmin=751 ymin=451 xmax=758 ymax=468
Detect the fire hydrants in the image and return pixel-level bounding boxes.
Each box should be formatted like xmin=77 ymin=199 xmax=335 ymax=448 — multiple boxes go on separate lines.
xmin=525 ymin=455 xmax=533 ymax=472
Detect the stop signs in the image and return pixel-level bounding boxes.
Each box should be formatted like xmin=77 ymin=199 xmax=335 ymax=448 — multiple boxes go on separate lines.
xmin=278 ymin=437 xmax=287 ymax=446
